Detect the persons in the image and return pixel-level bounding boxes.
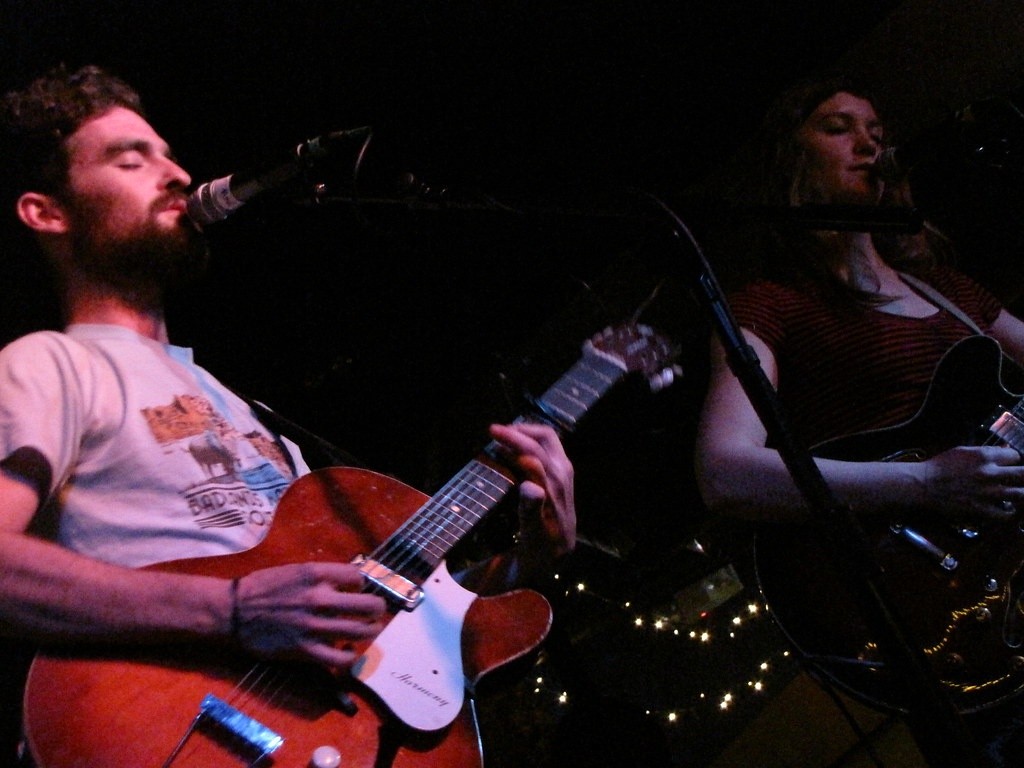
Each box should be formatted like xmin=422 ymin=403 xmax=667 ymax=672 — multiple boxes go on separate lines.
xmin=1 ymin=66 xmax=576 ymax=768
xmin=694 ymin=78 xmax=1024 ymax=522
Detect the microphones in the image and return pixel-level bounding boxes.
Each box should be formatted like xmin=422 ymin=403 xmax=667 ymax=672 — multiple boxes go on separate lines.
xmin=873 ymin=98 xmax=999 ymax=186
xmin=185 ymin=126 xmax=374 ymax=226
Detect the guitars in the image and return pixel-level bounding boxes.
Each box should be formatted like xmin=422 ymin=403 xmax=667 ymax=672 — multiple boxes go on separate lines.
xmin=736 ymin=329 xmax=1024 ymax=721
xmin=13 ymin=317 xmax=683 ymax=766
